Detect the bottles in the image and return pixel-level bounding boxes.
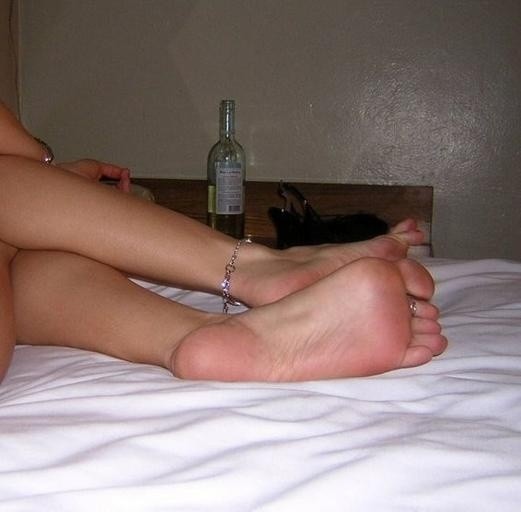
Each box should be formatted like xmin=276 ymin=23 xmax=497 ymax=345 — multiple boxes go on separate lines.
xmin=206 ymin=98 xmax=246 ymax=238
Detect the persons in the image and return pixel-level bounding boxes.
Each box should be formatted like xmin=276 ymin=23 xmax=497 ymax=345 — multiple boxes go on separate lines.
xmin=2 ymin=101 xmax=450 ymax=391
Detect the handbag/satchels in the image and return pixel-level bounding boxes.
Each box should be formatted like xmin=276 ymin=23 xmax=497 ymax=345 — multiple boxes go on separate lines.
xmin=268 ymin=182 xmax=388 ymax=250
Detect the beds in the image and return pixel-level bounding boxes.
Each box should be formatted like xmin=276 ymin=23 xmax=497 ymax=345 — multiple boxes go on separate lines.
xmin=0 ymin=177 xmax=521 ymax=512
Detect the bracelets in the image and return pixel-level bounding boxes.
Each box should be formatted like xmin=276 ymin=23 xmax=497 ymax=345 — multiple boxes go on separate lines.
xmin=33 ymin=137 xmax=54 ymax=167
